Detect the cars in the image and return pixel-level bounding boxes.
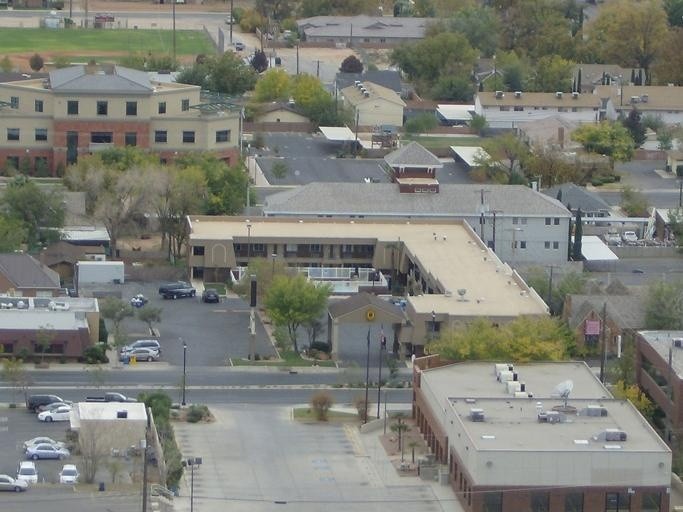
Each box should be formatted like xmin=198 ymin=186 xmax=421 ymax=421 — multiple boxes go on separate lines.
xmin=0 ymin=392 xmax=139 ymax=492
xmin=232 ymin=42 xmax=246 ymax=50
xmin=78 ymin=352 xmax=109 ymax=364
xmin=604 ymin=230 xmax=638 ymax=246
xmin=159 ymin=280 xmax=196 ymax=300
xmin=120 ymin=340 xmax=161 ymax=362
xmin=224 ymin=16 xmax=235 ymax=24
xmin=202 ymin=288 xmax=219 ymax=303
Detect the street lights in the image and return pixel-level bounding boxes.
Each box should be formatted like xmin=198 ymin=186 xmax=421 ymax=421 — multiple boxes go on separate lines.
xmin=272 ymin=253 xmax=277 ymax=273
xmin=181 ymin=457 xmax=202 ymax=512
xmin=247 ymin=224 xmax=252 ymax=263
